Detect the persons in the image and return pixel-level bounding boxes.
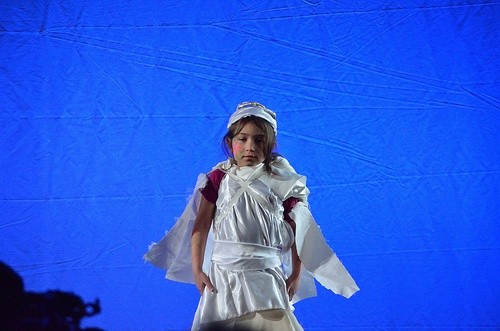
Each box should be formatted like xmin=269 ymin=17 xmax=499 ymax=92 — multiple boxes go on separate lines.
xmin=141 ymin=101 xmax=360 ymax=331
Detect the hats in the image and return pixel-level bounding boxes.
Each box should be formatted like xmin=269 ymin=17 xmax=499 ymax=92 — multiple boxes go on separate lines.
xmin=226 ymin=101 xmax=278 ymax=136
